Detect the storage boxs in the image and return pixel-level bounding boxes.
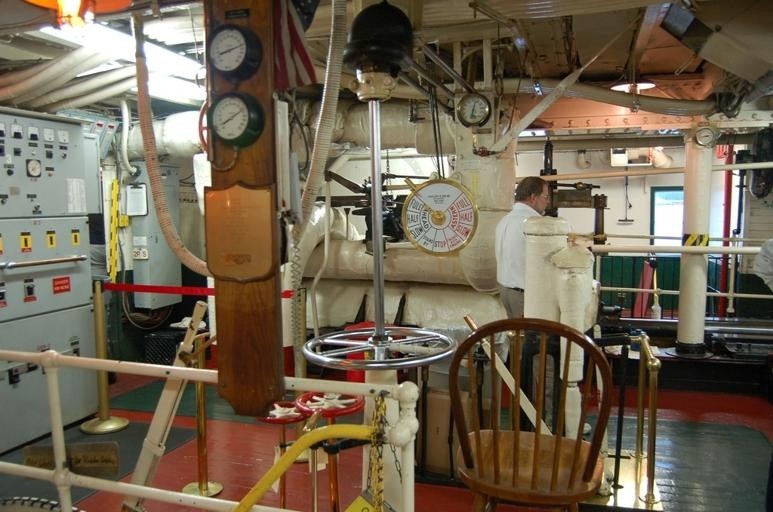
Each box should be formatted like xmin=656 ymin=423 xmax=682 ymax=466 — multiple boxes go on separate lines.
xmin=414 ymin=387 xmax=470 ymax=478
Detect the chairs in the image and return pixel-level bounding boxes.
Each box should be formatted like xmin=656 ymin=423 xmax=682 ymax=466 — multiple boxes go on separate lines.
xmin=448 ymin=317 xmax=616 ymax=512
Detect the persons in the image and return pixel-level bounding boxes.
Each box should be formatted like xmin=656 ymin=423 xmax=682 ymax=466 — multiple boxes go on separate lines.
xmin=492 ymin=176 xmax=595 ymax=437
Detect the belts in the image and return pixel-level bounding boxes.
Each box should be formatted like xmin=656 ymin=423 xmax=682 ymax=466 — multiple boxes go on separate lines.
xmin=507 ymin=287 xmax=524 ymax=293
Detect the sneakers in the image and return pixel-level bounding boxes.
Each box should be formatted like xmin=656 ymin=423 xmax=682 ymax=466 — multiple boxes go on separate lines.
xmin=583 ymin=423 xmax=591 ymax=435
